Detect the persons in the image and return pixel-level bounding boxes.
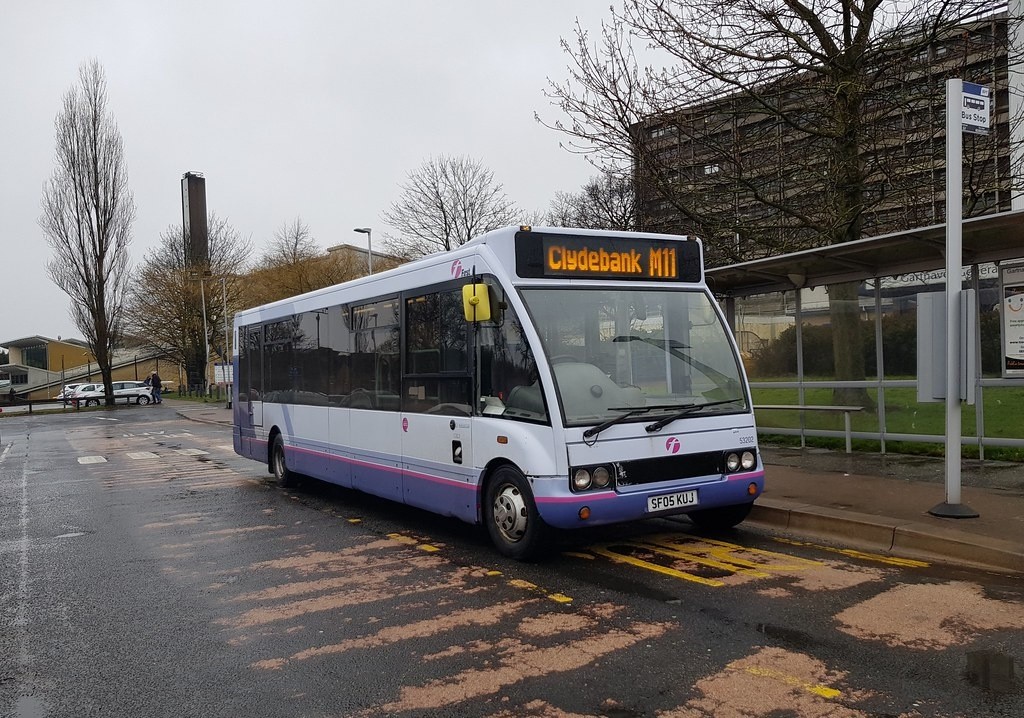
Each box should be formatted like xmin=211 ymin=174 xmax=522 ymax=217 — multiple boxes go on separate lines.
xmin=145 ymin=376 xmax=151 ymax=385
xmin=150 ymin=370 xmax=162 ymax=404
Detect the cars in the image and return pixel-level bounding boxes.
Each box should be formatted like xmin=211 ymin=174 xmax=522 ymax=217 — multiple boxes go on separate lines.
xmin=55 ymin=381 xmax=154 ymax=408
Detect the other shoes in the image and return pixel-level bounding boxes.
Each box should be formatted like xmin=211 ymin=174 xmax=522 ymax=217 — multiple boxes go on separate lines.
xmin=153 ymin=401 xmax=156 ymax=403
xmin=157 ymin=401 xmax=161 ymax=403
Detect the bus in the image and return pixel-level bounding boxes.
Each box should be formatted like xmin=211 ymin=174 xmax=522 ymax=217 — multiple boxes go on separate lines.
xmin=232 ymin=224 xmax=765 ymax=563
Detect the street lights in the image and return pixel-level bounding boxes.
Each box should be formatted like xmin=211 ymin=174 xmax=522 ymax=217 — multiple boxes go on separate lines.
xmin=83 ymin=352 xmax=94 ymax=383
xmin=353 ymin=227 xmax=373 ymax=276
xmin=218 ymin=277 xmax=232 ymax=409
